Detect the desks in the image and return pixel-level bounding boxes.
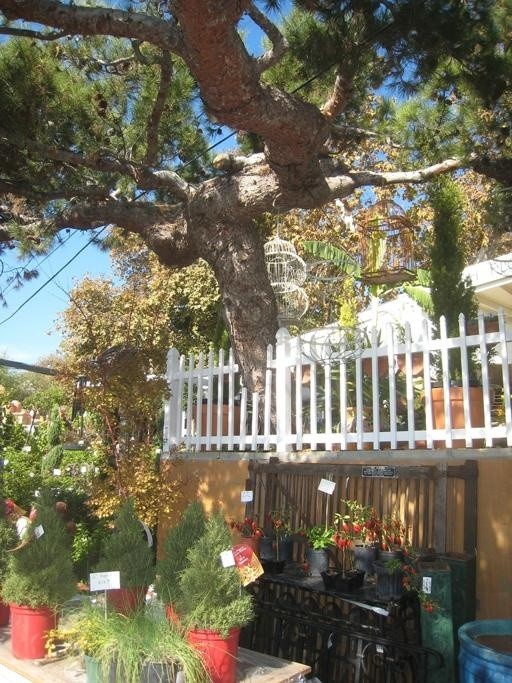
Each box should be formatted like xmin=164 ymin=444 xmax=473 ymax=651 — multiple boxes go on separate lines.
xmin=2 ymin=619 xmax=313 ymax=682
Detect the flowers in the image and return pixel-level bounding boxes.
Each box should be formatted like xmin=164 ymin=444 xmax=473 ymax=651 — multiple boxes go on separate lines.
xmin=229 ymin=500 xmax=444 ymax=613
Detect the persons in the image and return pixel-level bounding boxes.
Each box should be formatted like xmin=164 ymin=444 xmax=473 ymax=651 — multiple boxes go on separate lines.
xmin=7 ymin=510 xmax=32 ymax=541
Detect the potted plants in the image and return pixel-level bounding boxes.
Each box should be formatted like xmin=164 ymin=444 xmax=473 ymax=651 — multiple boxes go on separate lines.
xmin=1 ymin=490 xmax=259 ymax=683
xmin=293 ymin=353 xmax=420 ymax=433
